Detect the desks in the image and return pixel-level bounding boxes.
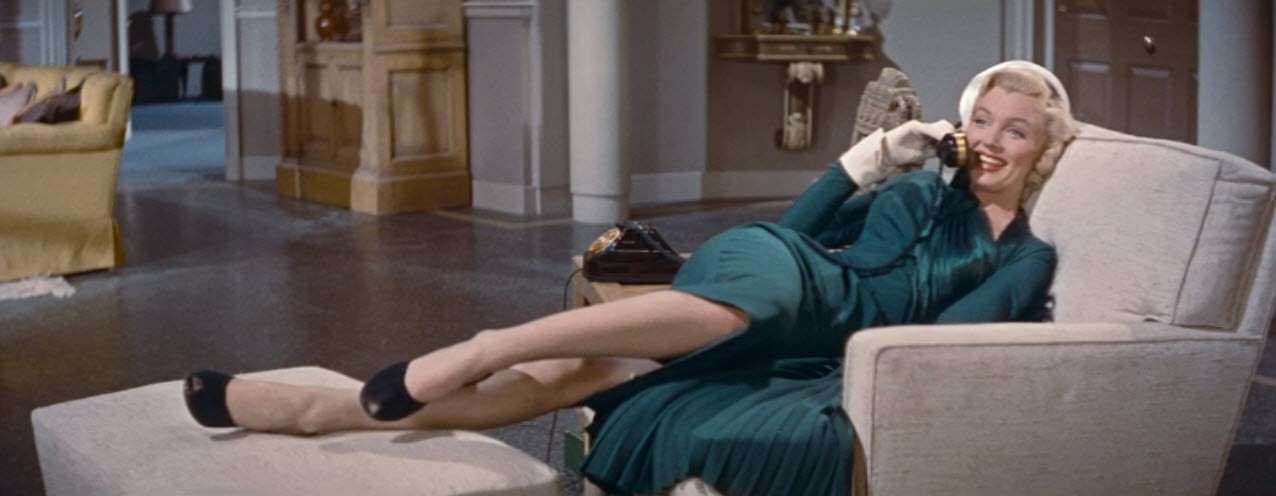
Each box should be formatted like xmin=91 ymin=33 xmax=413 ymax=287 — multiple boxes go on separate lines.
xmin=132 ymin=57 xmax=213 ymax=105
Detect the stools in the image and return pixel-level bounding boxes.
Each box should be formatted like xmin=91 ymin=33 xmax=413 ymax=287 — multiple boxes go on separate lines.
xmin=31 ymin=365 xmax=562 ymax=496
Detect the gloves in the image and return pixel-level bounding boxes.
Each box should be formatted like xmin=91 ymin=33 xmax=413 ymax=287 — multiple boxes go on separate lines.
xmin=838 ymin=119 xmax=956 ymax=193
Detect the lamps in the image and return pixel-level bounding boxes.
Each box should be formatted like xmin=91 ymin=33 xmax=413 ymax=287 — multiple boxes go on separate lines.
xmin=150 ymin=0 xmax=194 ymax=61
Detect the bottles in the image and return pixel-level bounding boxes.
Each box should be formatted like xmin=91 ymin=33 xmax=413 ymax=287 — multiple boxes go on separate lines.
xmin=315 ymin=0 xmax=351 ymax=44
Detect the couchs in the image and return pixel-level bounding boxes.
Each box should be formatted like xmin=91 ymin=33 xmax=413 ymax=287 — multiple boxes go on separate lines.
xmin=0 ymin=61 xmax=133 ymax=281
xmin=578 ymin=120 xmax=1276 ymax=496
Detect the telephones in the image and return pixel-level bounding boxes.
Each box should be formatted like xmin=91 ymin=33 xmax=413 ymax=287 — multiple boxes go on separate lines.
xmin=932 ymin=121 xmax=969 ymax=168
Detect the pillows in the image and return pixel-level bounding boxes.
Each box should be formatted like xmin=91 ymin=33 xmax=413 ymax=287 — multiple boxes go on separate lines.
xmin=0 ymin=75 xmax=86 ymax=126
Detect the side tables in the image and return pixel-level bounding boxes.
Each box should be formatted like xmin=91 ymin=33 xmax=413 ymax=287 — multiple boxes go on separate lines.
xmin=571 ymin=252 xmax=693 ymax=310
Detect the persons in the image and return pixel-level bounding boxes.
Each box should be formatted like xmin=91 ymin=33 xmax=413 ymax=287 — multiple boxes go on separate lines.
xmin=181 ymin=60 xmax=1076 ymax=496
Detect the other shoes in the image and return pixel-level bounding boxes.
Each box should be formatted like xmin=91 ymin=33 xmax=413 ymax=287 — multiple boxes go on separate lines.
xmin=184 ymin=370 xmax=236 ymax=427
xmin=358 ymin=357 xmax=493 ymax=422
